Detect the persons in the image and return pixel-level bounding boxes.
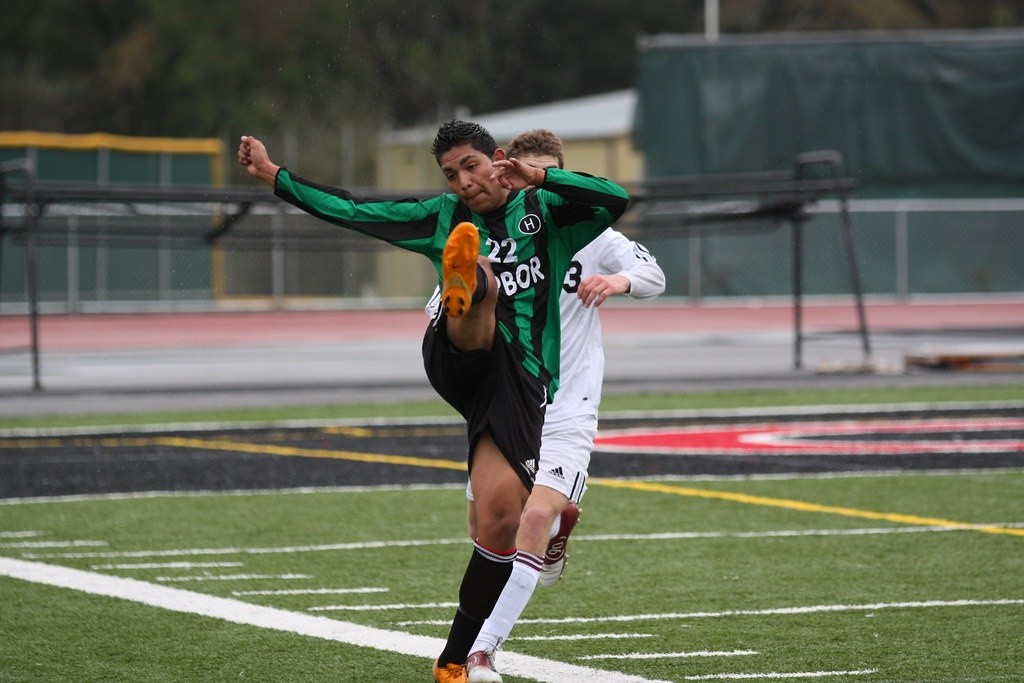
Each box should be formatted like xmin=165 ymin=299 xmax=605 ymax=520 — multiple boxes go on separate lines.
xmin=236 ymin=121 xmax=629 ymax=683
xmin=425 ymin=130 xmax=668 ymax=682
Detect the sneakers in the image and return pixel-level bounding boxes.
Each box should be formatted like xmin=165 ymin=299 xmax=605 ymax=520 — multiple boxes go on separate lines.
xmin=540 ymin=500 xmax=582 ymax=587
xmin=442 ymin=221 xmax=480 ymax=319
xmin=467 ymin=636 xmax=502 ymax=683
xmin=432 ymin=658 xmax=469 ymax=683
